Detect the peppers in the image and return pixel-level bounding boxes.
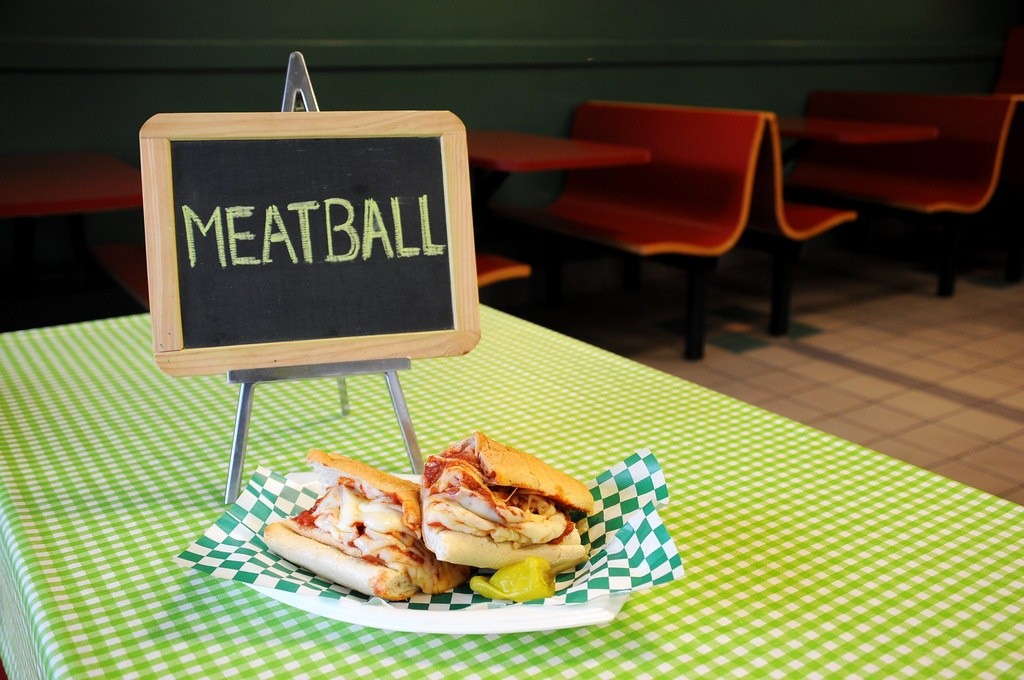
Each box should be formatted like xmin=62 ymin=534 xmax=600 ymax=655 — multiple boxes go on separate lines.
xmin=469 ymin=555 xmax=590 ymax=603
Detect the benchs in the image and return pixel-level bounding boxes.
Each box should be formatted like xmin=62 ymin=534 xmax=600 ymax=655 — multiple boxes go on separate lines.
xmin=741 ymin=111 xmax=859 ymax=338
xmin=785 ymin=89 xmax=1015 ymax=297
xmin=475 ymin=250 xmax=532 ymax=309
xmin=102 ymin=245 xmax=150 ymax=310
xmin=486 ymin=99 xmax=768 ymax=358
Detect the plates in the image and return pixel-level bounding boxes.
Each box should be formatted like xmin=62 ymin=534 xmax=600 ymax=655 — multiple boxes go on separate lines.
xmin=241 ymin=581 xmax=630 ymax=634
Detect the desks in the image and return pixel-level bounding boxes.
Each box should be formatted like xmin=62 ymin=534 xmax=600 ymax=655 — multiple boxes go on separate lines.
xmin=0 ymin=150 xmax=144 ymax=326
xmin=775 ymin=111 xmax=942 ymax=177
xmin=466 ymin=127 xmax=668 ymax=207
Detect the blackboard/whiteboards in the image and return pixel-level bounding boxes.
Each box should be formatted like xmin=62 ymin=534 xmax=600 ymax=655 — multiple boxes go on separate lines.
xmin=138 ymin=110 xmax=481 ymax=379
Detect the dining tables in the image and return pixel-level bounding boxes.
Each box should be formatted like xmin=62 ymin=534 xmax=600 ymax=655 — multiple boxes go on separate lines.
xmin=0 ymin=305 xmax=1024 ymax=680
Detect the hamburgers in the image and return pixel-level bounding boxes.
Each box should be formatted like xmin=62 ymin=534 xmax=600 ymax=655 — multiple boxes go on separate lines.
xmin=418 ymin=431 xmax=595 ymax=574
xmin=262 ymin=450 xmax=474 ymax=603
